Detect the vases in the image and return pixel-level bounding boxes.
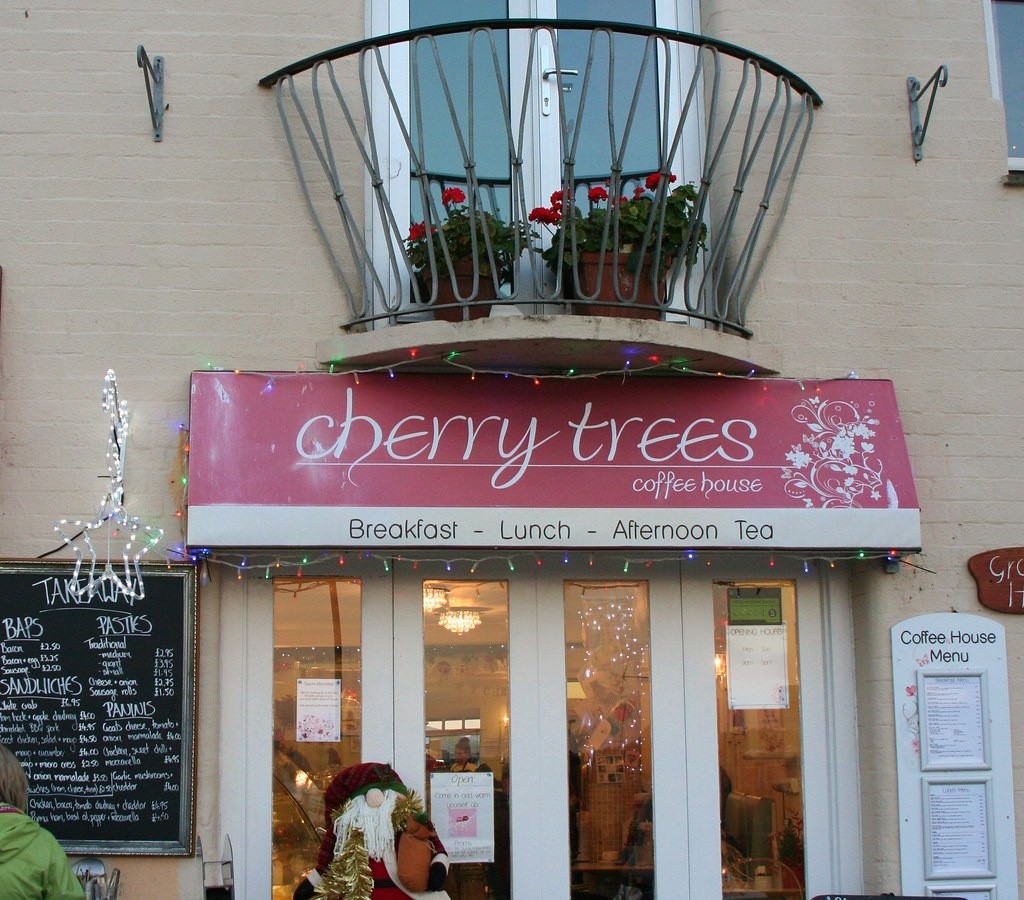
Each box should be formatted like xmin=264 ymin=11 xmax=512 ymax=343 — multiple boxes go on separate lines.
xmin=567 ymin=250 xmax=672 ymax=321
xmin=422 ymin=261 xmax=500 ymax=322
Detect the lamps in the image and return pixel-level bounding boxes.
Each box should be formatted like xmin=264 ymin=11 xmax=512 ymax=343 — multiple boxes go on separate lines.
xmin=438 ymin=607 xmax=483 ymax=635
xmin=423 ymin=583 xmax=451 ymax=613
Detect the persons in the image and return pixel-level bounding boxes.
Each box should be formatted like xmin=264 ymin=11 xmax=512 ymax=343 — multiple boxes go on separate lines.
xmin=616 ymin=766 xmax=730 ymax=867
xmin=570 ymin=751 xmax=581 ymax=862
xmin=0 ymin=744 xmax=87 ymax=900
xmin=292 ymin=762 xmax=450 ymax=900
xmin=449 ymin=737 xmax=510 ymax=900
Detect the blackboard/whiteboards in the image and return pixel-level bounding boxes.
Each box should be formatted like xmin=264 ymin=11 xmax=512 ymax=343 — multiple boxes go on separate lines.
xmin=0 ymin=557 xmax=199 ymax=856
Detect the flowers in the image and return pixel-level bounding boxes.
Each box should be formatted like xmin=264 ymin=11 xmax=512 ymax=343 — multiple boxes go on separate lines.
xmin=402 ymin=188 xmax=542 ymax=275
xmin=529 ymin=170 xmax=708 ymax=276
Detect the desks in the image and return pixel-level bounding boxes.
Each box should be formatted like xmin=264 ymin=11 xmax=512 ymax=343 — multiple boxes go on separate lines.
xmin=572 ymin=862 xmax=654 ymax=900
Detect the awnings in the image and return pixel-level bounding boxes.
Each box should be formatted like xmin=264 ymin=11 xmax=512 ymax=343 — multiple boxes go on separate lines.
xmin=186 ymin=370 xmax=921 ymax=558
